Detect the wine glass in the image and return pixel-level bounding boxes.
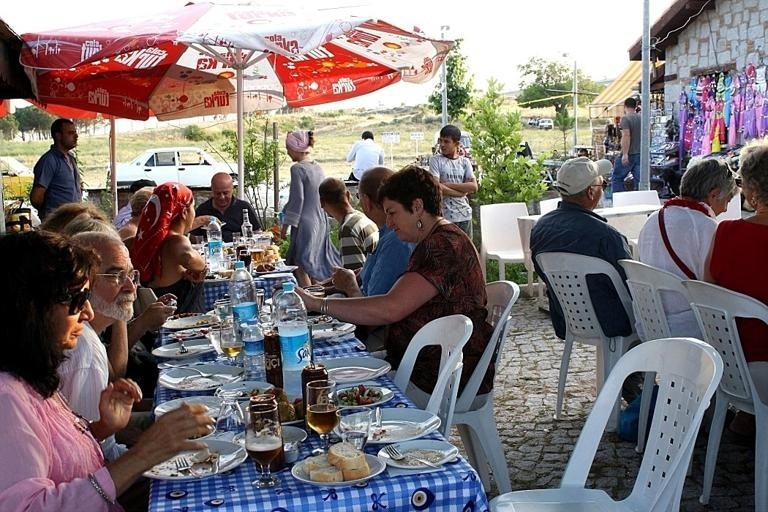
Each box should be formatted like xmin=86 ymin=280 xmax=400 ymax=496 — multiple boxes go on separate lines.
xmin=232 ymin=232 xmax=272 ymax=271
xmin=242 ymin=404 xmax=284 ymax=487
xmin=213 ymin=390 xmax=244 ymax=432
xmin=335 ymin=406 xmax=373 ymax=448
xmin=305 ymin=379 xmax=339 ymax=455
xmin=194 ymin=236 xmax=229 ymax=268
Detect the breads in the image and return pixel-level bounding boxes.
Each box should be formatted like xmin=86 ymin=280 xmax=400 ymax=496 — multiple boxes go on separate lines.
xmin=302 ymin=442 xmax=370 ymax=481
xmin=264 ymin=245 xmax=280 ymax=263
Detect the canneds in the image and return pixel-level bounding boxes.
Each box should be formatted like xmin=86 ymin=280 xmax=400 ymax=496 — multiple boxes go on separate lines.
xmin=250 ymin=394 xmax=285 ymax=471
xmin=264 ymin=330 xmax=283 ymax=388
xmin=302 ymin=362 xmax=328 ymax=424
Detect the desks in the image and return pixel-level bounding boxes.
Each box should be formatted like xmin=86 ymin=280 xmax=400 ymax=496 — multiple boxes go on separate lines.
xmin=517 ymin=204 xmax=662 ymax=296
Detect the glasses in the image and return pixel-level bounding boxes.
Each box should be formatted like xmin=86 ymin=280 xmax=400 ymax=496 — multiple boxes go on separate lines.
xmin=96 ymin=269 xmax=140 ymax=286
xmin=590 ymin=180 xmax=609 ymax=191
xmin=61 ymin=288 xmax=91 ymax=315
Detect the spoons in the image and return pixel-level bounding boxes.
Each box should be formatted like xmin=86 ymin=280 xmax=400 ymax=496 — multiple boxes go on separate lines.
xmin=374 ymin=404 xmax=385 ymax=436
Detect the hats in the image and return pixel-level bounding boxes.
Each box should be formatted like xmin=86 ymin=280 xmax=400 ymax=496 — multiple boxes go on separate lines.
xmin=556 ymin=156 xmax=612 ymax=195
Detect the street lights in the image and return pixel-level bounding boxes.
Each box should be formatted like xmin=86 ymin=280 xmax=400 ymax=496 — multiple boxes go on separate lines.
xmin=440 ymin=22 xmax=450 ymax=129
xmin=562 ymin=53 xmax=578 ymax=146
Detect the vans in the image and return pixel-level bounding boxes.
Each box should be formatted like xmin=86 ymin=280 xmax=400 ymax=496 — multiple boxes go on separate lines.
xmin=434 ymin=131 xmax=475 ymax=156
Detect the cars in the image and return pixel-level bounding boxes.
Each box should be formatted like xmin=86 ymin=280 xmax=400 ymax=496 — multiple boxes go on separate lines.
xmin=1 ymin=155 xmax=36 ymax=200
xmin=105 ymin=146 xmax=254 ymax=192
xmin=538 ymin=118 xmax=555 ymax=129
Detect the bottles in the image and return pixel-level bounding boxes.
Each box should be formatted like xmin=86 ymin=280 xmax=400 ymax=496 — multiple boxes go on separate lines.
xmin=230 ymin=260 xmax=261 ymax=364
xmin=206 ymin=216 xmax=223 ymax=254
xmin=240 ymin=208 xmax=253 ymax=237
xmin=276 ymin=281 xmax=312 ymax=401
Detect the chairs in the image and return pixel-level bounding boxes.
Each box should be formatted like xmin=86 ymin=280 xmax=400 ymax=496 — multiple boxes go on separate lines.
xmin=392 ymin=314 xmax=473 ymax=438
xmin=490 ymin=337 xmax=724 ymax=512
xmin=680 ymin=278 xmax=768 ymax=512
xmin=452 ymin=280 xmax=520 ymax=495
xmin=480 ymin=202 xmax=530 ymax=281
xmin=535 ymin=252 xmax=638 ymax=433
xmin=539 ymin=197 xmax=562 ymax=216
xmin=618 ymin=258 xmax=693 ymax=476
xmin=613 ymin=190 xmax=661 ymax=206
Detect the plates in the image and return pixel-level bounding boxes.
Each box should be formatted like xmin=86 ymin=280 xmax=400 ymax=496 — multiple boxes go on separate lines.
xmin=232 ymin=424 xmax=307 ymax=452
xmin=144 ymin=438 xmax=247 ymax=483
xmin=289 ymin=452 xmax=385 ymax=487
xmin=158 ymin=365 xmax=245 ymax=392
xmin=329 ymin=385 xmax=395 ymax=407
xmin=236 ymin=401 xmax=304 ymax=425
xmin=150 ymin=289 xmax=393 ymax=381
xmin=378 ymin=438 xmax=459 ymax=469
xmin=332 ymin=406 xmax=442 ymax=443
xmin=217 ymin=379 xmax=275 ymax=400
xmin=252 ymin=267 xmax=280 ymax=274
xmin=153 ymin=396 xmax=222 ymax=420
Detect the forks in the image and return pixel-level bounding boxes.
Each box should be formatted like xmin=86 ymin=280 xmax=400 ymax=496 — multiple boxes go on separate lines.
xmin=385 ymin=444 xmax=440 ymax=470
xmin=174 ymin=457 xmax=202 ymax=481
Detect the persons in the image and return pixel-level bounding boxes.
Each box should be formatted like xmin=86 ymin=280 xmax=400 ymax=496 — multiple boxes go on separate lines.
xmin=611 ymin=99 xmax=652 ymax=192
xmin=280 ymin=129 xmax=343 ymax=288
xmin=29 ymin=120 xmax=82 ymax=224
xmin=0 ymin=167 xmax=496 ymax=512
xmin=347 ymin=130 xmax=384 ymax=181
xmin=617 ymin=160 xmax=739 ymax=446
xmin=530 ymin=157 xmax=656 ymax=403
xmin=703 ymin=139 xmax=768 ymax=445
xmin=429 ymin=125 xmax=481 ymax=251
